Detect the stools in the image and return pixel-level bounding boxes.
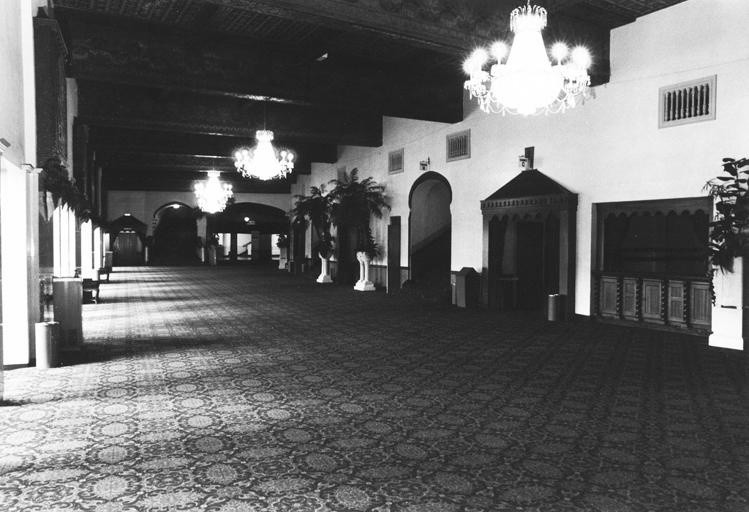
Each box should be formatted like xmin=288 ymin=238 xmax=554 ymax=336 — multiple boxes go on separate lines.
xmin=82 ymin=280 xmax=100 ymax=304
xmin=98 ymin=267 xmax=109 ymax=283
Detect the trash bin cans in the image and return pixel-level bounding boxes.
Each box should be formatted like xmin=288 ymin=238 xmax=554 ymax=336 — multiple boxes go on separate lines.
xmin=457 ymin=267 xmax=477 ymax=307
xmin=216 ymin=244 xmax=224 ymax=265
xmin=53 ymin=278 xmax=83 ymax=352
xmin=105 ymin=252 xmax=113 ymax=272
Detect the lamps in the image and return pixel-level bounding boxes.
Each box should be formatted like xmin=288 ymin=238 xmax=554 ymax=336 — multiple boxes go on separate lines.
xmin=460 ymin=0 xmax=593 ymax=117
xmin=192 ymin=159 xmax=233 ymax=214
xmin=232 ymin=107 xmax=294 ymax=182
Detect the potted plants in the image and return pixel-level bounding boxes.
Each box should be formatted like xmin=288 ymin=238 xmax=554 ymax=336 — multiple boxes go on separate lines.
xmin=286 ymin=168 xmax=391 ymax=291
